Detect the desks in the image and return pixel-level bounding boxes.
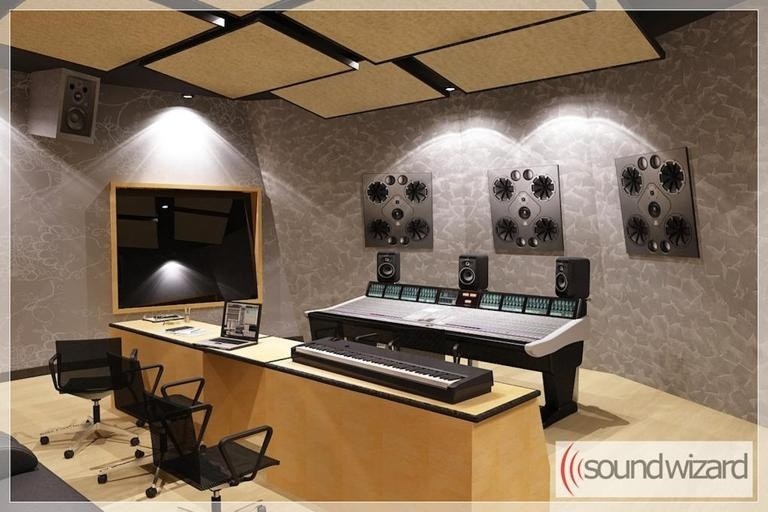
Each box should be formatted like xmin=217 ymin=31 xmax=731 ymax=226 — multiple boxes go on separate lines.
xmin=106 ymin=315 xmax=553 ymax=507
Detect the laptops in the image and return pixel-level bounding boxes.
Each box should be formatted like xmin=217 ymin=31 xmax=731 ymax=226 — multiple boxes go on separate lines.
xmin=191 ymin=301 xmax=262 ymax=350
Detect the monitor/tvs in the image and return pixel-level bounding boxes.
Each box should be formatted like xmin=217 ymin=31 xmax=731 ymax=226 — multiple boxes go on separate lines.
xmin=109 ymin=182 xmax=263 ymax=315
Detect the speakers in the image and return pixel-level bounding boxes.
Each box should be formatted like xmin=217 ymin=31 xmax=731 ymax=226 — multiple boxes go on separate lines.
xmin=555 ymin=257 xmax=591 ymax=299
xmin=27 ymin=67 xmax=101 ymax=145
xmin=459 ymin=255 xmax=489 ymax=290
xmin=377 ymin=250 xmax=401 ymax=283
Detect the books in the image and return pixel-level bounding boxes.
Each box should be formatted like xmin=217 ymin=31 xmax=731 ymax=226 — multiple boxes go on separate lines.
xmin=167 ymin=326 xmax=207 ymax=336
xmin=142 ymin=314 xmax=184 ymax=323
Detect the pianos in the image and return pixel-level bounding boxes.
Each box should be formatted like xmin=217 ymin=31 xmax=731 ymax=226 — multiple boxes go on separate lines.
xmin=291 ymin=336 xmax=494 ymax=404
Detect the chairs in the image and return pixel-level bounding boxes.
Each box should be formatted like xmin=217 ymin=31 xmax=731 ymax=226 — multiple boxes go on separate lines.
xmin=39 ymin=334 xmax=274 ymax=510
